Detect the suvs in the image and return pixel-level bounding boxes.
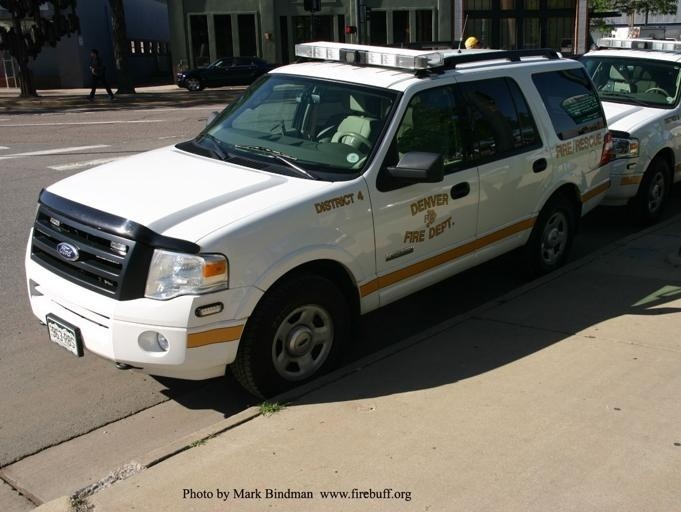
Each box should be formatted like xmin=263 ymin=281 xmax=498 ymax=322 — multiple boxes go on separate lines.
xmin=579 ymin=33 xmax=681 ymax=227
xmin=23 ymin=12 xmax=616 ymax=403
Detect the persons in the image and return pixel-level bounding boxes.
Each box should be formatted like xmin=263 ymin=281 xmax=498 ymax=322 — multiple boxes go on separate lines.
xmin=485 ymin=42 xmax=500 ymax=50
xmin=560 ymin=39 xmax=573 ymax=53
xmin=86 ymin=48 xmax=116 ymax=104
xmin=464 ymin=36 xmax=481 ymax=50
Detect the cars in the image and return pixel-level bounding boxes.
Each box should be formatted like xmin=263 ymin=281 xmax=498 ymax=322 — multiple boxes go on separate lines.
xmin=174 ymin=54 xmax=283 ymax=89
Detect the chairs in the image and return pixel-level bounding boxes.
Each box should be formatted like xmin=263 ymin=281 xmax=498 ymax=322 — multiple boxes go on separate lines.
xmin=602 ymin=64 xmax=661 ymax=93
xmin=331 ymin=91 xmax=450 ymax=164
